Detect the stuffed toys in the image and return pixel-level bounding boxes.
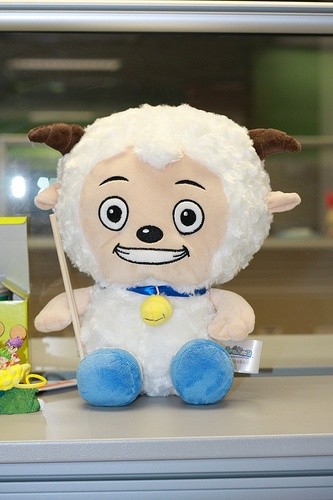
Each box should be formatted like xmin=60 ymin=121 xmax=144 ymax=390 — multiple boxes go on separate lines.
xmin=29 ymin=102 xmax=303 ymax=403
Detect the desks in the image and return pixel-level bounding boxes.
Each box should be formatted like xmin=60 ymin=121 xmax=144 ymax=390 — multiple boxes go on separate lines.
xmin=0 ymin=335 xmax=333 ymax=500
